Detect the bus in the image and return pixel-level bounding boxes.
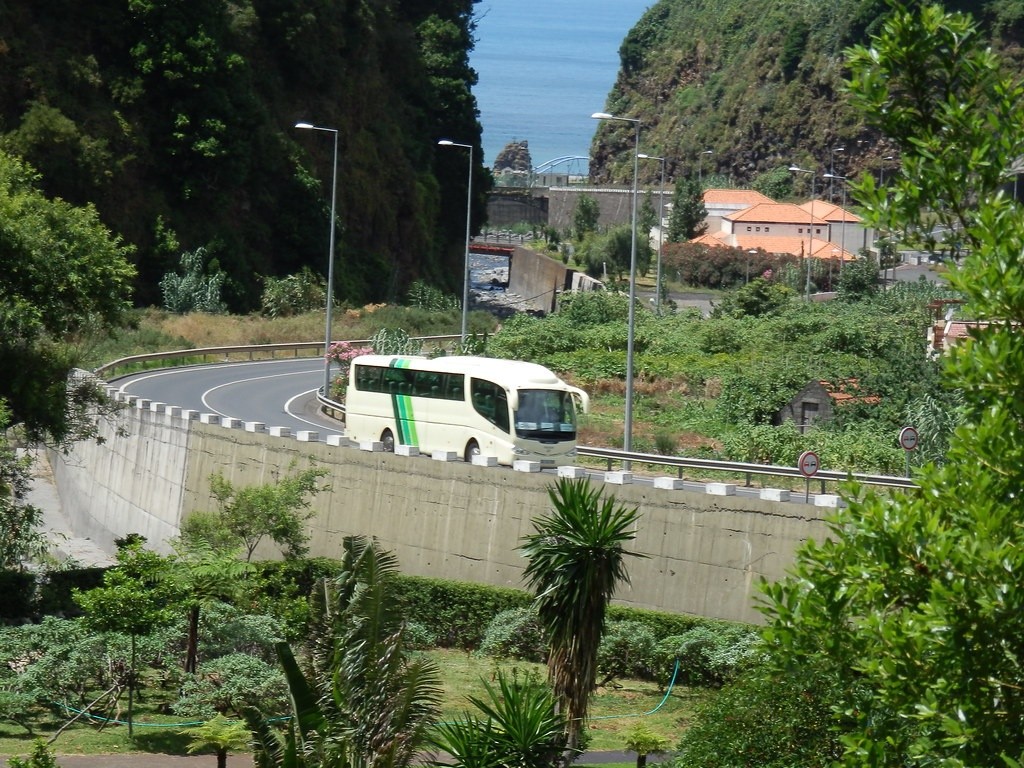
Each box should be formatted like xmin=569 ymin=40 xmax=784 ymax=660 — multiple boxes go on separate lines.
xmin=343 ymin=353 xmax=592 ymax=472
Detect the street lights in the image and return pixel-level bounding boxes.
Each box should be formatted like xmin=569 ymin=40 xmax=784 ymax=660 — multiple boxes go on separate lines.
xmin=637 ymin=153 xmax=665 ymax=314
xmin=829 ymin=146 xmax=845 ymax=202
xmin=294 ymin=122 xmax=339 ymax=400
xmin=879 ymin=155 xmax=892 ymax=188
xmin=823 ymin=172 xmax=847 ymax=294
xmin=437 ymin=140 xmax=473 ymax=353
xmin=592 ymin=112 xmax=641 ymax=470
xmin=789 ymin=165 xmax=817 ymax=303
